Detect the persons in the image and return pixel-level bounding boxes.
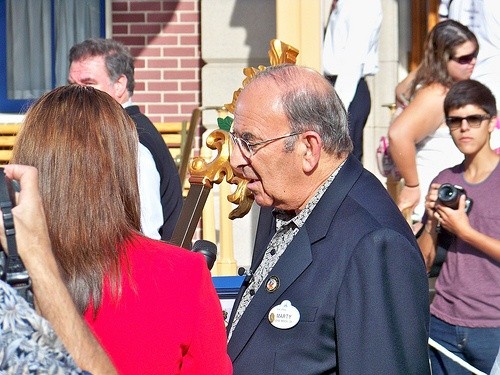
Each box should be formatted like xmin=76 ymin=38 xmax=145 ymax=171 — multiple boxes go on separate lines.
xmin=396 ymin=0 xmax=500 ymax=155
xmin=389 ymin=20 xmax=480 ymax=237
xmin=68 ymin=38 xmax=184 ymax=243
xmin=414 ymin=79 xmax=500 ymax=375
xmin=321 ymin=0 xmax=384 ymax=161
xmin=226 ymin=66 xmax=431 ymax=375
xmin=0 ymin=84 xmax=234 ymax=375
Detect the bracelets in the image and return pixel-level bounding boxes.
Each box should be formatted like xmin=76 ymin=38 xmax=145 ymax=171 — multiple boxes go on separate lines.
xmin=405 ymin=184 xmax=419 ymax=188
xmin=425 ymin=223 xmax=440 ymax=235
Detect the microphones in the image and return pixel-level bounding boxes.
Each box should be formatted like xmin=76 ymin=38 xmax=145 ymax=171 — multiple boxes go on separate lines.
xmin=191 ymin=240 xmax=217 ymax=271
xmin=238 ymin=267 xmax=252 ymax=277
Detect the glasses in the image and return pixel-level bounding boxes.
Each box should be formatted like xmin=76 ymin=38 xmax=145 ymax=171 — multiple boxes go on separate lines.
xmin=446 ymin=114 xmax=492 ymax=128
xmin=227 ymin=131 xmax=308 ymax=158
xmin=449 ymin=46 xmax=480 ymax=64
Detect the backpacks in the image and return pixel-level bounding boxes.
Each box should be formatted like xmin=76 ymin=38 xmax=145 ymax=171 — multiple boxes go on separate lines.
xmin=377 ymin=80 xmax=430 ymax=181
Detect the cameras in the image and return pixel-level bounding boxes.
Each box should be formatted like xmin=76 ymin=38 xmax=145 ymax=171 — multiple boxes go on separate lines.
xmin=435 ymin=182 xmax=473 ymax=215
